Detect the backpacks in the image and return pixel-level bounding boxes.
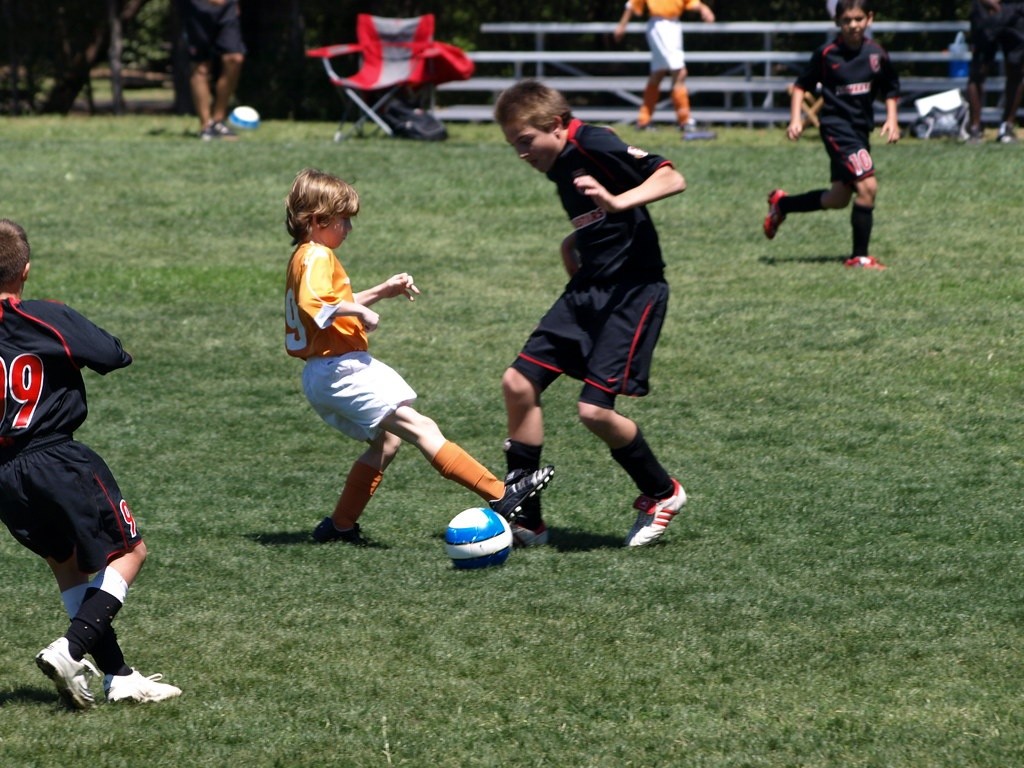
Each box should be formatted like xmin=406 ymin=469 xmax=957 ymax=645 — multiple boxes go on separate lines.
xmin=383 ymin=99 xmax=447 ymax=141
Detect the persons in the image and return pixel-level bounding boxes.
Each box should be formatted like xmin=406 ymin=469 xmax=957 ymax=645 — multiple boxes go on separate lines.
xmin=170 ymin=0 xmax=246 ymax=140
xmin=272 ymin=167 xmax=558 ymax=540
xmin=762 ymin=0 xmax=899 ymax=271
xmin=0 ymin=217 xmax=184 ymax=711
xmin=963 ymin=0 xmax=1024 ymax=143
xmin=614 ymin=0 xmax=719 ymax=139
xmin=488 ymin=77 xmax=692 ymax=549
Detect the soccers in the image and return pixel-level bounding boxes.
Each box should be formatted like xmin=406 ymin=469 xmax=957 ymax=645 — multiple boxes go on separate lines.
xmin=228 ymin=105 xmax=260 ymax=129
xmin=445 ymin=507 xmax=514 ymax=569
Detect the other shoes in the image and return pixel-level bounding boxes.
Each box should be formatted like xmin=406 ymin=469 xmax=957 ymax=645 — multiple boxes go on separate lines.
xmin=200 ymin=123 xmax=215 ymax=142
xmin=213 ymin=121 xmax=237 ymax=140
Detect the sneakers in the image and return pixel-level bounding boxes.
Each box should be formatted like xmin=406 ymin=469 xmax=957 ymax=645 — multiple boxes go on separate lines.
xmin=311 ymin=516 xmax=386 ymax=549
xmin=35 ymin=637 xmax=100 ymax=710
xmin=685 ymin=130 xmax=716 ymax=143
xmin=625 ymin=478 xmax=688 ymax=549
xmin=104 ymin=667 xmax=182 ymax=703
xmin=845 ymin=255 xmax=886 ymax=271
xmin=763 ymin=188 xmax=786 ymax=240
xmin=509 ymin=521 xmax=548 ymax=548
xmin=486 ymin=465 xmax=555 ymax=521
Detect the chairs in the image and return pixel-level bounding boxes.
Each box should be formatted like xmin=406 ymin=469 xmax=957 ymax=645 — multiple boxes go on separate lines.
xmin=305 ymin=15 xmax=474 ymax=143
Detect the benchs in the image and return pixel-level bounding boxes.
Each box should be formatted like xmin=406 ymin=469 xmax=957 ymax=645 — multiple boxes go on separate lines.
xmin=427 ymin=22 xmax=1024 ymax=125
